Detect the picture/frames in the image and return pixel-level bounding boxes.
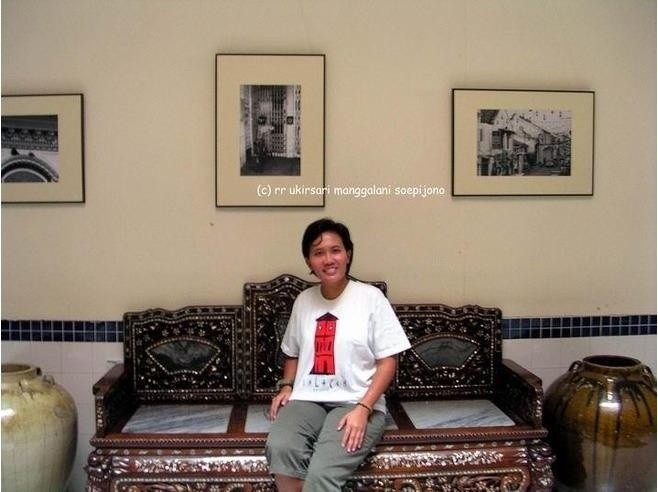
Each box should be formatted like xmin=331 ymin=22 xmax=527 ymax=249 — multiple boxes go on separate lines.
xmin=2 ymin=94 xmax=85 ymax=203
xmin=452 ymin=88 xmax=595 ymax=197
xmin=215 ymin=52 xmax=326 ymax=207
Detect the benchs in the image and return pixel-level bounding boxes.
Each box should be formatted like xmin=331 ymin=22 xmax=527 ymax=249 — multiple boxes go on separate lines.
xmin=83 ymin=273 xmax=557 ymax=492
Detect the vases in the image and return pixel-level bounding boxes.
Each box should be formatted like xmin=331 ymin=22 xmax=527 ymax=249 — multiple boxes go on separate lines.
xmin=542 ymin=354 xmax=657 ymax=491
xmin=2 ymin=363 xmax=78 ymax=492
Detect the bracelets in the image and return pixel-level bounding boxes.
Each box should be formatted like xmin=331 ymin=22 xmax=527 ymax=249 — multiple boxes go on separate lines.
xmin=356 ymin=402 xmax=373 ymax=414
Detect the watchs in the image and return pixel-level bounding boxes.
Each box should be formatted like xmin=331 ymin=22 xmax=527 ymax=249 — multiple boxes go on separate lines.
xmin=275 ymin=379 xmax=293 ymax=391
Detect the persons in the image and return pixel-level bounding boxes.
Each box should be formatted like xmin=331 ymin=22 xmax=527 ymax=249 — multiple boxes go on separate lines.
xmin=265 ymin=218 xmax=412 ymax=492
